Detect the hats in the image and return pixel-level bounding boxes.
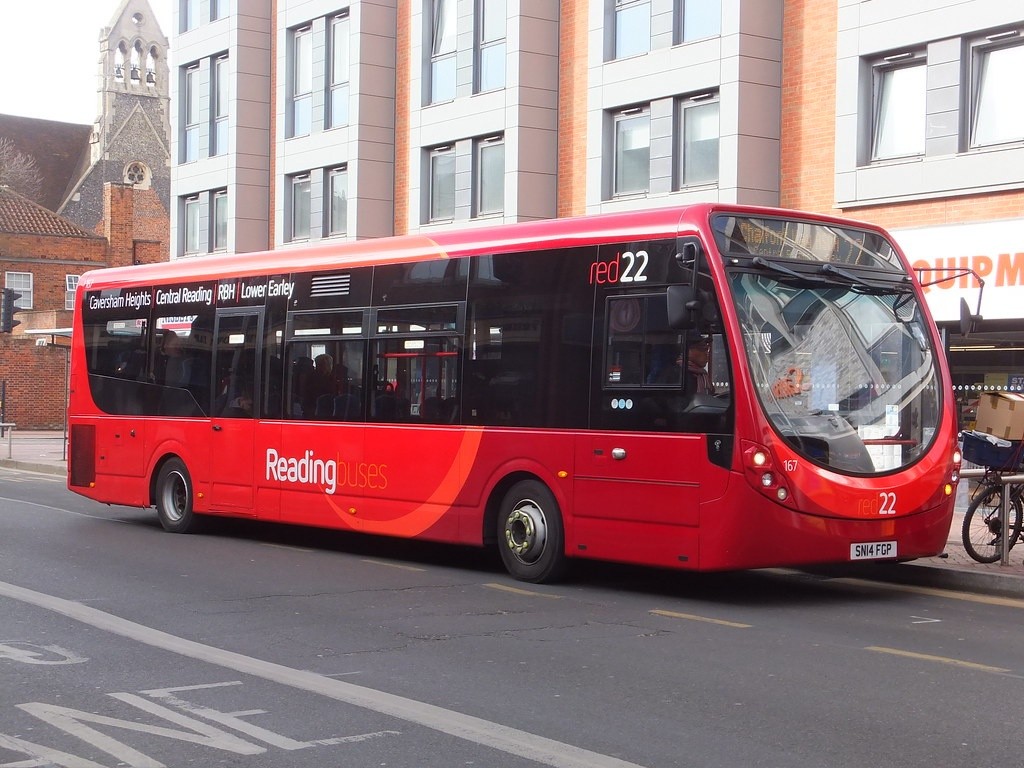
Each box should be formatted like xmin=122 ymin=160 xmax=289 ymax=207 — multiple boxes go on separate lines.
xmin=683 ymin=329 xmax=714 ymax=346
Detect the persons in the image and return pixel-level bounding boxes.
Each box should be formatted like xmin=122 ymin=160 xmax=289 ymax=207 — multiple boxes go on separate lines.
xmin=654 ymin=329 xmax=715 ymax=413
xmin=152 ymin=331 xmax=341 ymax=420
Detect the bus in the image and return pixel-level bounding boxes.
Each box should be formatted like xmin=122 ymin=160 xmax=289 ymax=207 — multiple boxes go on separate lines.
xmin=67 ymin=200 xmax=985 ymax=583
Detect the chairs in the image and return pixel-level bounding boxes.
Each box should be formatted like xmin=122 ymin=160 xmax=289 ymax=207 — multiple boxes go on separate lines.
xmin=179 ymin=355 xmax=200 ymax=390
xmin=119 ymin=348 xmax=147 ymax=382
xmin=313 ymin=394 xmax=445 ymax=421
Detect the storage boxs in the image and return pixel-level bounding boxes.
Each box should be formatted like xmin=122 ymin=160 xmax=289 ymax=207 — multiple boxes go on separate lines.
xmin=975 ymin=391 xmax=1024 ymax=440
xmin=961 ymin=429 xmax=1021 ymax=471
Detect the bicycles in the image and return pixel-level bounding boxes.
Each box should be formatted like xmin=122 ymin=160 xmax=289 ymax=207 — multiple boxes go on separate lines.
xmin=963 ymin=467 xmax=1023 ymax=564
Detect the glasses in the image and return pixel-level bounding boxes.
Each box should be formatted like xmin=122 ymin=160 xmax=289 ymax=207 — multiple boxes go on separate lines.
xmin=691 ymin=345 xmax=708 ymax=352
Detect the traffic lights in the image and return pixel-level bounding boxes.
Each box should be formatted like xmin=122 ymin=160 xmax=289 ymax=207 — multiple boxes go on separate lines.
xmin=0 ymin=287 xmax=23 ymax=333
